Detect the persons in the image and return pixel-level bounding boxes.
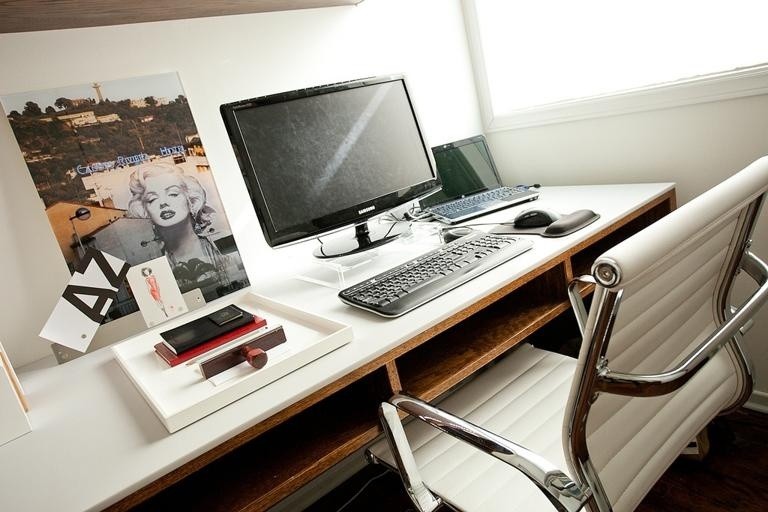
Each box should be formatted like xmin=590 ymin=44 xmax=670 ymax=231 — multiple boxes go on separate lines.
xmin=140 ymin=267 xmax=168 ymax=319
xmin=129 ymin=162 xmax=225 ymax=286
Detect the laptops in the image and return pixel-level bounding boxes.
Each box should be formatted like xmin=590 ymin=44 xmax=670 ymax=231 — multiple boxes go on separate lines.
xmin=419 ymin=134 xmax=540 ymax=225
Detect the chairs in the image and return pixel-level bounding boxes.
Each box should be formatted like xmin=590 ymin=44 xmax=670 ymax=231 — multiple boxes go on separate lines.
xmin=368 ymin=157 xmax=768 ymax=510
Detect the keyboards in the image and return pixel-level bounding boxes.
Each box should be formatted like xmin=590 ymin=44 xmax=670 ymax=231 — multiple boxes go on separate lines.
xmin=337 ymin=229 xmax=536 ymax=318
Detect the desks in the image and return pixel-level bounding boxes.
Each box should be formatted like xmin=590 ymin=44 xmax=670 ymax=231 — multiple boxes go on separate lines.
xmin=0 ymin=183 xmax=709 ymax=512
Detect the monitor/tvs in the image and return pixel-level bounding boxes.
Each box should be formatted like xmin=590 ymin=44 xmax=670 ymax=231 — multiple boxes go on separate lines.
xmin=219 ymin=70 xmax=444 ymax=259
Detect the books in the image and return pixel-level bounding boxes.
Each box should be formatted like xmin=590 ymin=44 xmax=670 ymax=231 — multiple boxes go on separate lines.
xmin=154 ymin=314 xmax=267 ymax=367
xmin=161 ymin=305 xmax=255 ymax=355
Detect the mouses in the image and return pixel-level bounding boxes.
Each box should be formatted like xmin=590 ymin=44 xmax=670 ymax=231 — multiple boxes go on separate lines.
xmin=515 ymin=208 xmax=556 ymax=231
